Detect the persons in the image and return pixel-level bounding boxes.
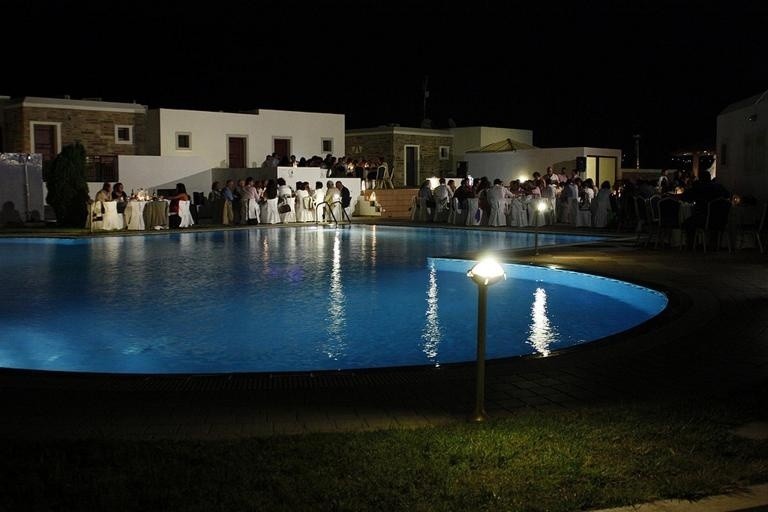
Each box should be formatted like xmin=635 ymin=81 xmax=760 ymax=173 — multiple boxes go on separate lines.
xmin=209 ymin=177 xmax=351 ymax=226
xmin=96 ymin=182 xmax=128 ymax=213
xmin=418 ymin=166 xmax=726 ymax=231
xmin=262 ymin=153 xmax=393 ymax=189
xmin=163 ymin=182 xmax=188 ymax=216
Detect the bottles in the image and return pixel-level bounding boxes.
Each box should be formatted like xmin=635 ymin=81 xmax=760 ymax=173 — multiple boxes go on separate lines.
xmin=131 ymin=188 xmax=134 ymax=197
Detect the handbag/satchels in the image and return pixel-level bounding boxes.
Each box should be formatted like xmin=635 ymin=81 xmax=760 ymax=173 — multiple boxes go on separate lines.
xmin=247 ymin=218 xmax=258 ymax=226
xmin=279 ymin=205 xmax=291 ymax=213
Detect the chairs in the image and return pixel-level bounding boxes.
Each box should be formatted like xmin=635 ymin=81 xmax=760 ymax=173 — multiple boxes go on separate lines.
xmin=410 ymin=182 xmax=767 ymax=257
xmin=87 ymin=194 xmax=349 ymax=230
xmin=279 ymin=159 xmax=396 ymax=191
